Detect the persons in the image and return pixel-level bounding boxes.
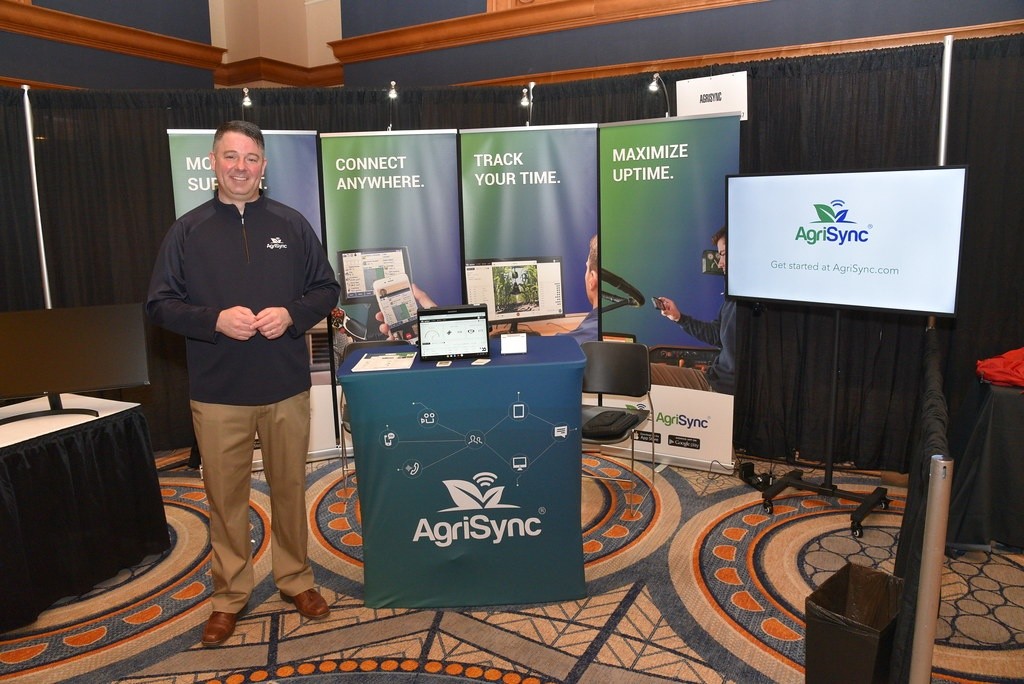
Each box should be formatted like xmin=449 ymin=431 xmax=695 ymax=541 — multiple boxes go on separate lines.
xmin=650 ymin=226 xmax=734 ymax=397
xmin=554 ymin=233 xmax=598 ymax=344
xmin=375 ymin=283 xmax=438 ymax=341
xmin=145 ymin=120 xmax=339 ymax=647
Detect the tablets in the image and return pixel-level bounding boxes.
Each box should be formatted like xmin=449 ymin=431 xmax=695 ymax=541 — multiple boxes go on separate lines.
xmin=417 ymin=306 xmax=489 ymax=363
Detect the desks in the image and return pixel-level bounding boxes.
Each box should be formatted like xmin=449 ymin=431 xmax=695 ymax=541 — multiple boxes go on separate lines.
xmin=0 ymin=390 xmax=172 ymax=634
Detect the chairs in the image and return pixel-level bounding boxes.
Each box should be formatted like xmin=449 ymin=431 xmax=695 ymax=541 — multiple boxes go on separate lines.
xmin=580 ymin=342 xmax=655 ymax=517
xmin=339 ymin=340 xmax=412 ymax=504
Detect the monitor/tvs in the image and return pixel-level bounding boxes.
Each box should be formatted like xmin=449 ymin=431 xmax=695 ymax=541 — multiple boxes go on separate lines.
xmin=0 ymin=300 xmax=150 ymax=426
xmin=337 ymin=246 xmax=413 ymax=305
xmin=724 ymin=165 xmax=970 ymax=316
xmin=465 ymin=256 xmax=565 ymax=337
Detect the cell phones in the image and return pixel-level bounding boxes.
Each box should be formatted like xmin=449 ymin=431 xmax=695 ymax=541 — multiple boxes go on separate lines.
xmin=652 ymin=297 xmax=666 ymax=311
xmin=373 ymin=273 xmax=420 ymax=345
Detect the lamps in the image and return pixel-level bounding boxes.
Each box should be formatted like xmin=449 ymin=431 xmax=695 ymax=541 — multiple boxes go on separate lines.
xmin=241 ymin=87 xmax=252 ymax=122
xmin=649 ymin=72 xmax=670 ymax=119
xmin=387 ymin=81 xmax=397 ymax=131
xmin=520 ymin=81 xmax=536 ymax=126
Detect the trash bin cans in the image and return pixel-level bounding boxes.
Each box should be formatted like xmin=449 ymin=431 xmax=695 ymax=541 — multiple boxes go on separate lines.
xmin=805 ymin=564 xmax=903 ymax=684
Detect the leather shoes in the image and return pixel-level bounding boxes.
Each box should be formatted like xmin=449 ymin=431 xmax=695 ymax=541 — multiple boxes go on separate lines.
xmin=202 ymin=603 xmax=249 ymax=646
xmin=280 ymin=588 xmax=330 ymax=619
